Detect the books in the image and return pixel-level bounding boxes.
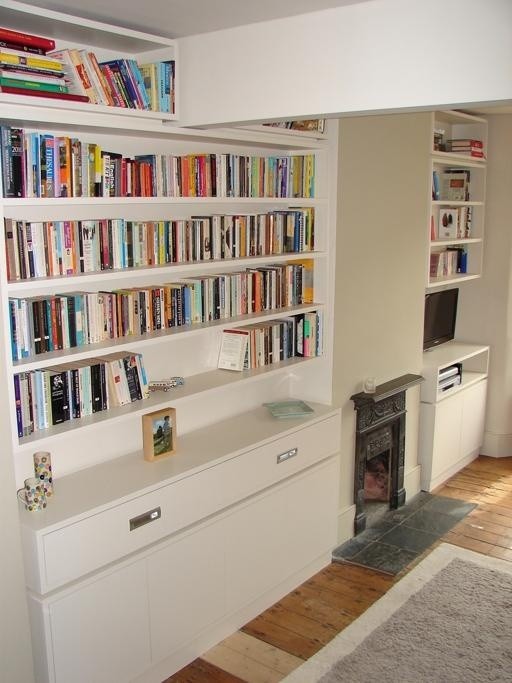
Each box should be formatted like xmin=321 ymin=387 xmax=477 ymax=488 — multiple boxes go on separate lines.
xmin=217 ymin=311 xmax=324 ymax=371
xmin=1 ymin=29 xmax=175 ymax=114
xmin=14 ymin=351 xmax=150 ymax=436
xmin=9 ymin=259 xmax=315 ymax=362
xmin=4 ymin=207 xmax=315 ymax=282
xmin=428 ymin=126 xmax=487 ymax=284
xmin=1 ymin=118 xmax=326 ymax=199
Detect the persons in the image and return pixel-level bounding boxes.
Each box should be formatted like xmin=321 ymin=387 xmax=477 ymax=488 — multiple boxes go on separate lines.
xmin=162 ymin=415 xmax=172 ymax=446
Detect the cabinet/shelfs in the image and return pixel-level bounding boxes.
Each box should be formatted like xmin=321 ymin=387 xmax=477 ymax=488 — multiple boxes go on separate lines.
xmin=419 ymin=345 xmax=490 ymax=493
xmin=424 ymin=110 xmax=489 ymax=288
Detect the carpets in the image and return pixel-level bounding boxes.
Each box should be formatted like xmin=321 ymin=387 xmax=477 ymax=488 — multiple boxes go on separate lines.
xmin=277 ymin=542 xmax=512 ymax=683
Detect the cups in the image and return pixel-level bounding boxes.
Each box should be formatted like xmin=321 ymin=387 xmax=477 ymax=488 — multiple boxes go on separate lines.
xmin=16 ymin=478 xmax=46 ymax=511
xmin=34 ymin=452 xmax=54 ymax=496
